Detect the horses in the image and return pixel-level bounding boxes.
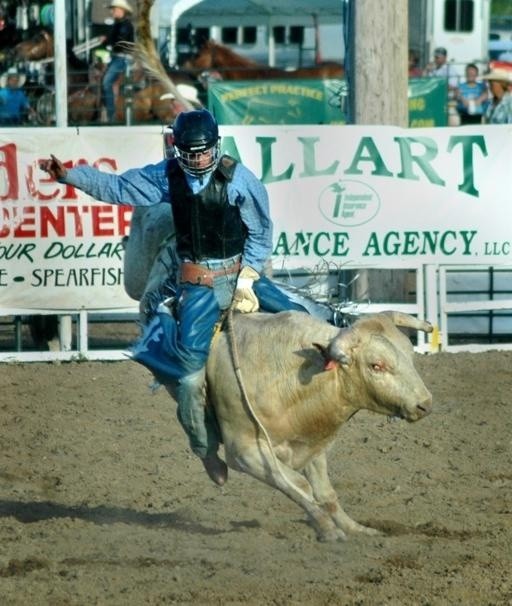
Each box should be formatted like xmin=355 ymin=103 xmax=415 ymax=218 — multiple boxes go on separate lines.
xmin=185 ymin=38 xmax=344 ymax=80
xmin=22 ymin=26 xmax=193 ymax=121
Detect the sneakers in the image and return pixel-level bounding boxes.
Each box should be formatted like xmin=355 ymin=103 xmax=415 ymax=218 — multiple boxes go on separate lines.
xmin=202 ymin=452 xmax=227 ymax=485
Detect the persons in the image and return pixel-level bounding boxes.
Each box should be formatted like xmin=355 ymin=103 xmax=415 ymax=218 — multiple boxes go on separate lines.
xmin=480 ymin=69 xmax=512 ymax=124
xmin=1 ymin=67 xmax=38 ymax=126
xmin=452 ymin=62 xmax=489 ymax=124
xmin=93 ymin=0 xmax=135 ymax=124
xmin=421 ymin=47 xmax=458 ymax=101
xmin=39 ymin=108 xmax=353 ymax=487
xmin=408 ymin=46 xmax=422 ymax=77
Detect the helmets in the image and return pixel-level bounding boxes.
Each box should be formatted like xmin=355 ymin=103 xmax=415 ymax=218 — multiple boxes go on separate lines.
xmin=172 ymin=108 xmax=221 ymax=178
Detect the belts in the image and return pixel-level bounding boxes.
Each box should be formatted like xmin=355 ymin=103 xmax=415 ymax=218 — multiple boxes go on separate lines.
xmin=182 ymin=261 xmax=240 ymax=286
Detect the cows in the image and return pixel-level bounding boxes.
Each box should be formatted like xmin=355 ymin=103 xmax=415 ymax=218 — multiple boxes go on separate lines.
xmin=123 ymin=35 xmax=436 ymax=542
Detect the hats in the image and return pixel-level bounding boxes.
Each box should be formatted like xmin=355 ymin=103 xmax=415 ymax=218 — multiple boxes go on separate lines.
xmin=0 ymin=67 xmax=26 ymax=88
xmin=431 ymin=48 xmax=446 ymax=57
xmin=159 ymin=84 xmax=202 ymax=107
xmin=475 ymin=68 xmax=512 ymax=83
xmin=102 ymin=0 xmax=133 ymax=16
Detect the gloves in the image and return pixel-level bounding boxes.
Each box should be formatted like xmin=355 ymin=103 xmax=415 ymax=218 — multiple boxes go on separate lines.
xmin=231 ymin=266 xmax=259 ymax=313
